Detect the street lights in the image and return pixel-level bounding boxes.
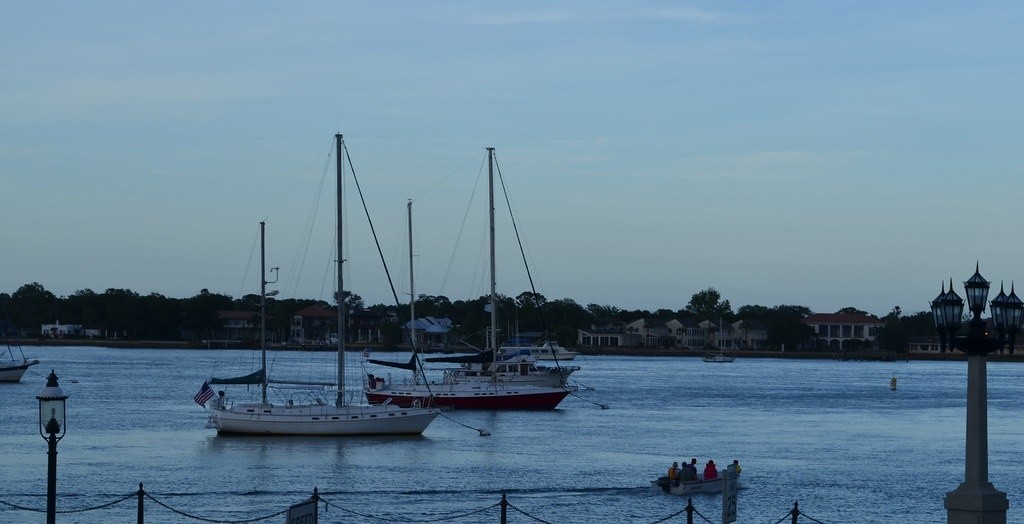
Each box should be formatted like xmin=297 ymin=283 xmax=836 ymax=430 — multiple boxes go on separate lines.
xmin=926 ymin=258 xmax=1024 ymax=524
xmin=35 ymin=370 xmax=68 ymax=524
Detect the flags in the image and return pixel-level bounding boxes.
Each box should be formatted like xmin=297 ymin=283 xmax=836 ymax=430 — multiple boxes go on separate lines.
xmin=363 ymin=348 xmax=370 ymax=357
xmin=194 ymin=380 xmax=215 ymax=408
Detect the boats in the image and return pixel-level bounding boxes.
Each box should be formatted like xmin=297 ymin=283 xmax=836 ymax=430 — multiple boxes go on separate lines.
xmin=0 ymin=334 xmax=40 ymax=383
xmin=495 ymin=340 xmax=578 ymax=361
xmin=650 ymin=472 xmax=739 ymax=496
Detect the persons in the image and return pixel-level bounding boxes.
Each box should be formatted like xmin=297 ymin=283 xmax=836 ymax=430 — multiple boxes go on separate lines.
xmin=668 ymin=458 xmax=701 ymax=481
xmin=704 ymin=460 xmax=718 ymax=480
xmin=733 ymin=460 xmax=742 ymax=474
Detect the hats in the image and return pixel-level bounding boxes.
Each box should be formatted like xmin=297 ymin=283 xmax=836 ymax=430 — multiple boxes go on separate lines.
xmin=682 ymin=462 xmax=687 ymax=468
xmin=673 ymin=462 xmax=678 ymax=467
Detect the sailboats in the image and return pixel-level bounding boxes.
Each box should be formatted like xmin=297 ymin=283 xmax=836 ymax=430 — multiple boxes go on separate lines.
xmin=352 ymin=144 xmax=580 ymax=410
xmin=204 ymin=128 xmax=442 ymax=435
xmin=701 ymin=316 xmax=736 ymax=362
